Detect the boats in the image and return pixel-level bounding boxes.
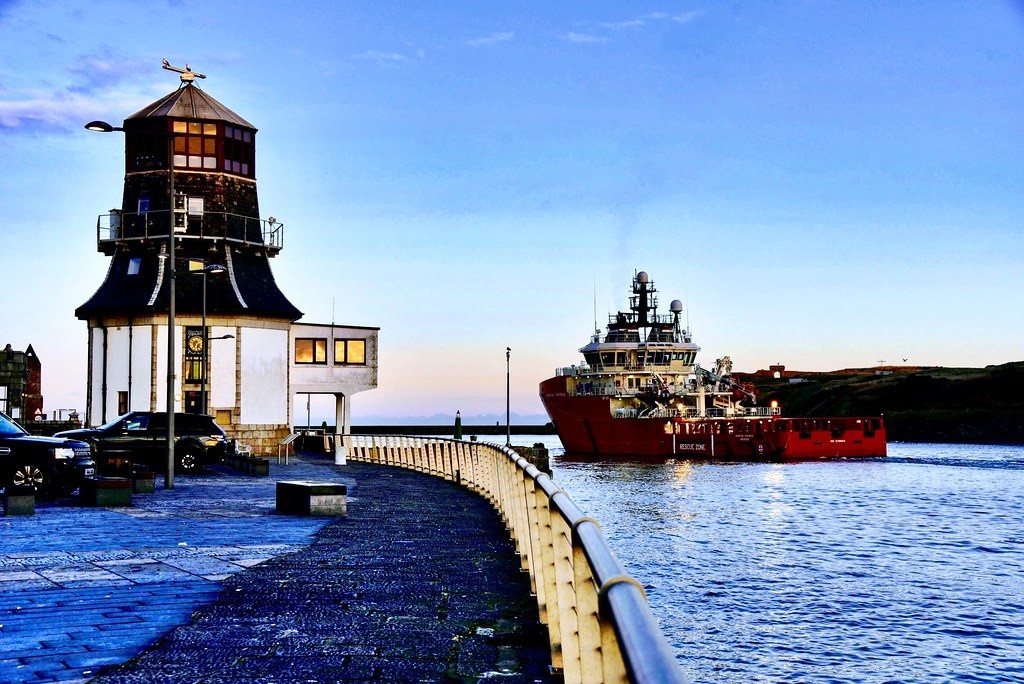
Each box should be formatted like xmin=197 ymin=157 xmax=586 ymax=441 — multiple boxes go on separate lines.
xmin=538 ymin=268 xmax=888 ymax=462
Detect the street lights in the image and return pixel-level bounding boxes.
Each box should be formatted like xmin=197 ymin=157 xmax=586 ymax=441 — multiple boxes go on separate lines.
xmin=86 ymin=121 xmax=176 ymax=490
xmin=201 ymin=265 xmax=236 ymax=414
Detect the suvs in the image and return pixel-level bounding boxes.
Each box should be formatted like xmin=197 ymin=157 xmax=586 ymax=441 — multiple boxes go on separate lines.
xmin=54 ymin=410 xmax=227 ymax=475
xmin=0 ymin=414 xmax=96 ymax=498
